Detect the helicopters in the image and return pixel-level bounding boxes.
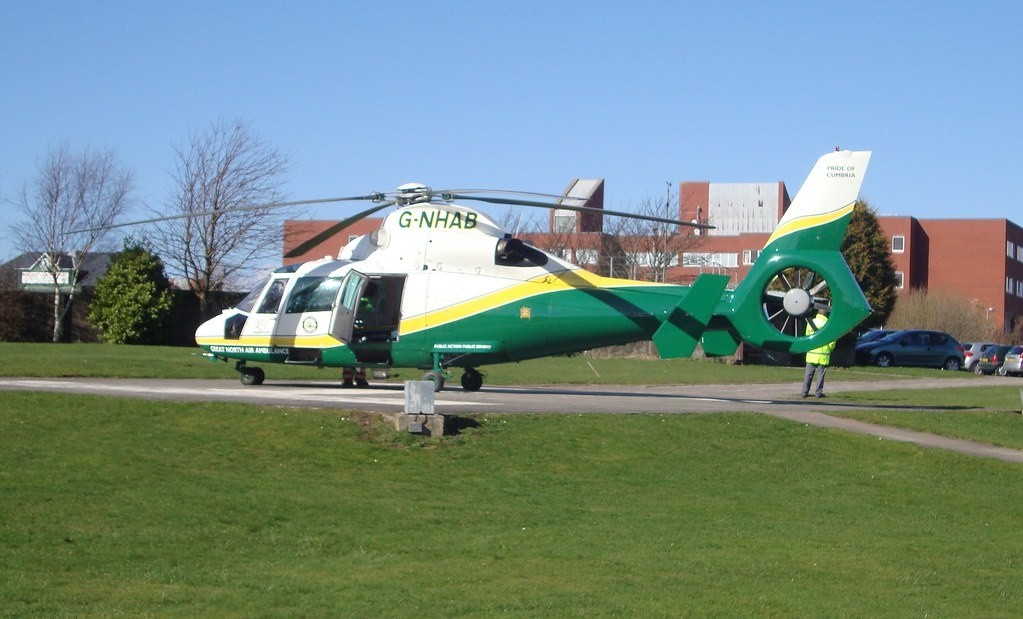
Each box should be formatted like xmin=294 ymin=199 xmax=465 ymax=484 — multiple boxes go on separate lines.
xmin=58 ymin=150 xmax=873 ymax=392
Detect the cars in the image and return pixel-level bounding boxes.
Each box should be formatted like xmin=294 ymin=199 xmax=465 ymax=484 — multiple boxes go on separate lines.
xmin=857 ymin=329 xmax=965 ymax=371
xmin=962 ymin=343 xmax=1023 ymax=376
xmin=855 ymin=329 xmax=897 ymax=343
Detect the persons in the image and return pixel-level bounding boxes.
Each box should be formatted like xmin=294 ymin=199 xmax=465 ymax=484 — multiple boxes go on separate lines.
xmin=341 ymin=366 xmax=369 ymax=387
xmin=801 ymin=308 xmax=836 ymax=399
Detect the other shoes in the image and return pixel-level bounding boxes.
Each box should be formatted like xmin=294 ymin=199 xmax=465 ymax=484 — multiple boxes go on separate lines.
xmin=817 ymin=393 xmax=825 ymax=398
xmin=802 ymin=394 xmax=806 ymax=398
